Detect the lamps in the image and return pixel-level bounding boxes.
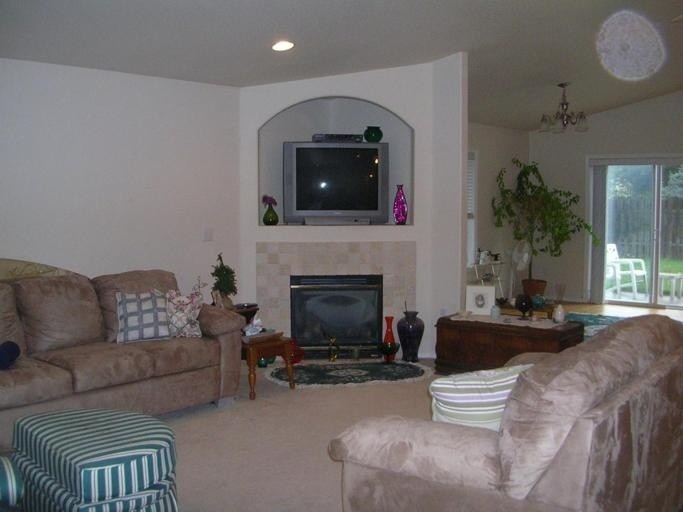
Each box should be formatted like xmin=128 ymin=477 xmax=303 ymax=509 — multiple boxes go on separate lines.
xmin=537 ymin=80 xmax=592 ymax=138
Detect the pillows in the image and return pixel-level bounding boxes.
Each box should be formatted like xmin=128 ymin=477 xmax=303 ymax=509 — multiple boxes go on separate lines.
xmin=112 ymin=287 xmax=169 ymax=345
xmin=159 ymin=287 xmax=206 ymax=340
xmin=423 ymin=362 xmax=533 ymax=432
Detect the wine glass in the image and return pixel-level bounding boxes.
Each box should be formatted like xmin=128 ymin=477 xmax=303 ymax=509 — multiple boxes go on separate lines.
xmin=515 ymin=294 xmax=530 ymax=320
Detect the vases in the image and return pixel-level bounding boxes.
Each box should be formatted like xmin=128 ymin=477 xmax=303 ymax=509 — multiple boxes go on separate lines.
xmin=362 ymin=125 xmax=383 ymax=143
xmin=262 ymin=204 xmax=278 ymax=226
xmin=378 ymin=315 xmax=400 ymax=363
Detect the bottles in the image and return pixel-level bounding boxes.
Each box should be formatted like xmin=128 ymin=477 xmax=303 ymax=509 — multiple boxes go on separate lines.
xmin=490 ymin=305 xmax=496 ymax=322
xmin=383 ymin=316 xmax=396 ymax=360
xmin=258 ymin=327 xmax=277 ymax=366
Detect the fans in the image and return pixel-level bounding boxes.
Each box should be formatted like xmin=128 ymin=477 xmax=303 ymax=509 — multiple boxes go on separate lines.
xmin=499 ymin=239 xmax=532 ymax=303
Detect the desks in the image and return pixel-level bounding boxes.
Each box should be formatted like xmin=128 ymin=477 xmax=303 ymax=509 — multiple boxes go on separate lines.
xmin=240 ymin=331 xmax=296 ymax=399
xmin=231 ymin=302 xmax=258 ymax=324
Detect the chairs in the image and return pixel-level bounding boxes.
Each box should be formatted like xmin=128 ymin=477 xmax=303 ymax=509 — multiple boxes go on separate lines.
xmin=601 ymin=241 xmax=649 ymax=303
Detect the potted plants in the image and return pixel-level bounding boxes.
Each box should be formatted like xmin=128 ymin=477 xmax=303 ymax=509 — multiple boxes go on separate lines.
xmin=485 ymin=154 xmax=595 ymax=300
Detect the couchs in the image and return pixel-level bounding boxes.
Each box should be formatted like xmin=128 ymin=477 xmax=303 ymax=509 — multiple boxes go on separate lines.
xmin=326 ymin=312 xmax=681 ymax=512
xmin=0 ymin=263 xmax=244 ymax=452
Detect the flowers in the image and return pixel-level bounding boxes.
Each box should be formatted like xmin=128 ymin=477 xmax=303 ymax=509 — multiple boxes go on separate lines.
xmin=259 ymin=192 xmax=278 ymax=206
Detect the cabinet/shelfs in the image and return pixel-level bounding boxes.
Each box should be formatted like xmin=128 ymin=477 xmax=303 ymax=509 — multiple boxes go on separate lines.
xmin=430 ymin=310 xmax=583 ymax=379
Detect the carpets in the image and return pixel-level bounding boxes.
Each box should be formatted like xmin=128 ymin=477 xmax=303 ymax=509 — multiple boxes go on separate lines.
xmin=565 ymin=311 xmax=630 ymax=341
xmin=260 ymin=356 xmax=433 ymax=391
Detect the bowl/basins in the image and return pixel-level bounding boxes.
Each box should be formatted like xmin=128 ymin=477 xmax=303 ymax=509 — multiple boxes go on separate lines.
xmin=233 ymin=303 xmax=258 ymax=324
xmin=379 ymin=342 xmax=399 ymax=355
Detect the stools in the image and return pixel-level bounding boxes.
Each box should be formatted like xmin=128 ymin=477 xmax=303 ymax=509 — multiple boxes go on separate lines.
xmin=0 ymin=408 xmax=181 ymax=511
xmin=653 ymin=272 xmax=682 ymax=302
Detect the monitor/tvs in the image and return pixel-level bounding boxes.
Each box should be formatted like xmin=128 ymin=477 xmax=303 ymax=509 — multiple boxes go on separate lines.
xmin=283 ymin=141 xmax=389 ymax=226
